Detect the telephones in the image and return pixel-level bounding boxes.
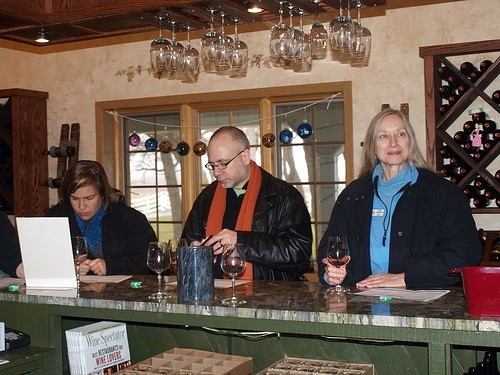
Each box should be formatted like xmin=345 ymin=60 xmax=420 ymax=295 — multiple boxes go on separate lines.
xmin=6 ymin=327 xmax=32 ymax=352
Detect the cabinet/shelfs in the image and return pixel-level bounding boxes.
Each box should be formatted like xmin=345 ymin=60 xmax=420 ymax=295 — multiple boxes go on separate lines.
xmin=418 ymin=39 xmax=500 ymax=269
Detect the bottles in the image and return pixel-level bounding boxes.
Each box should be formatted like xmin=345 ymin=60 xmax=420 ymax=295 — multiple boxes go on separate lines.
xmin=463 ymin=351 xmax=500 ymax=375
xmin=436 ymin=59 xmax=500 ymax=264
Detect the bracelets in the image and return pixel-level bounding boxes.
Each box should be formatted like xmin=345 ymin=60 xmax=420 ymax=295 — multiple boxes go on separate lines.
xmin=218 ymin=240 xmax=223 ymax=247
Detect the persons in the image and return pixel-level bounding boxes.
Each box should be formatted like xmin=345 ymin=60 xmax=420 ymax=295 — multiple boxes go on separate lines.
xmin=0 ymin=209 xmax=25 ymax=278
xmin=43 ymin=160 xmax=171 ymax=278
xmin=177 ymin=126 xmax=313 ymax=284
xmin=316 ymin=107 xmax=482 ymax=291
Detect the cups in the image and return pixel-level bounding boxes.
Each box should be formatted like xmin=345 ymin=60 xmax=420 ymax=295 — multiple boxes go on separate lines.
xmin=168 ymin=238 xmax=187 ymax=269
xmin=177 ymin=245 xmax=214 ymax=302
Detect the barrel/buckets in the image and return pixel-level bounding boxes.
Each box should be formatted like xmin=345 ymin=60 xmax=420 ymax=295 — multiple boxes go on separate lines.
xmin=449 ymin=267 xmax=500 ymax=317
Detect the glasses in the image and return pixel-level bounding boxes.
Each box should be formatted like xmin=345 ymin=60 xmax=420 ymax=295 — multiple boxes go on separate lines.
xmin=204 ymin=151 xmax=245 ymax=171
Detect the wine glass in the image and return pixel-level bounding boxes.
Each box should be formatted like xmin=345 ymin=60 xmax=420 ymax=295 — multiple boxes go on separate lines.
xmin=220 ymin=244 xmax=246 ymax=307
xmin=327 ymin=236 xmax=350 ymax=293
xmin=149 ymin=0 xmax=373 ymax=84
xmin=71 ymin=236 xmax=89 ymax=289
xmin=147 ymin=242 xmax=172 ymax=299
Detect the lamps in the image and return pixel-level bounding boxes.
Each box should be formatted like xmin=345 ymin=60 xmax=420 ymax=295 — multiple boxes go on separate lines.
xmin=34 ymin=27 xmax=50 ymax=43
